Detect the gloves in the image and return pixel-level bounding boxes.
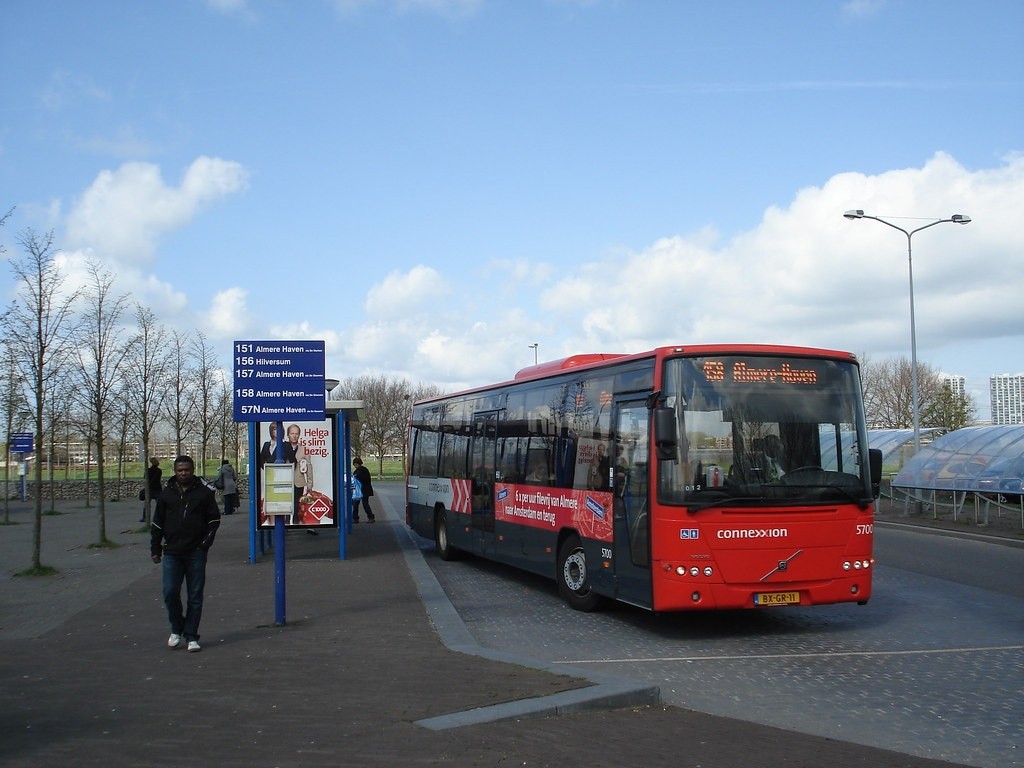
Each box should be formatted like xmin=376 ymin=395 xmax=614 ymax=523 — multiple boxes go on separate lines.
xmin=306 ymin=490 xmax=312 ymax=495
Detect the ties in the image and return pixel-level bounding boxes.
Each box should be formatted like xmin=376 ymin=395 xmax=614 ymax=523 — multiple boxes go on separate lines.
xmin=770 ymin=459 xmax=778 ymax=481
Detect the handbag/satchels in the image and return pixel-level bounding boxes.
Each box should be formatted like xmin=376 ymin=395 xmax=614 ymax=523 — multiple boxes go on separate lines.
xmin=139 ymin=488 xmax=145 ymax=501
xmin=350 ymin=475 xmax=363 ymax=501
xmin=215 ymin=474 xmax=224 ymax=489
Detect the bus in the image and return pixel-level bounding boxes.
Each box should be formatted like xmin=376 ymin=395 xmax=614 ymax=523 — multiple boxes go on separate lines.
xmin=401 ymin=343 xmax=883 ymax=617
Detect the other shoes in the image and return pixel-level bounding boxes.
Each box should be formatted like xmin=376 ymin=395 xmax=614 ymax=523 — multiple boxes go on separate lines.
xmin=222 ymin=511 xmax=232 ymax=515
xmin=307 ymin=529 xmax=318 ymax=535
xmin=188 ymin=641 xmax=200 ymax=652
xmin=168 ymin=634 xmax=180 ymax=647
xmin=368 ymin=518 xmax=375 ymax=523
xmin=352 ymin=519 xmax=359 ymax=523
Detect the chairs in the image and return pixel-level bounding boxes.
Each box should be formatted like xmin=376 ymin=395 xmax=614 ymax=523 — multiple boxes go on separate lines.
xmin=751 ymin=435 xmax=789 ymax=479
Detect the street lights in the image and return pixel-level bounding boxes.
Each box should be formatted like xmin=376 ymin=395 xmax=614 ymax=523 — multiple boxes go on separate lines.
xmin=528 ymin=344 xmax=538 ymax=364
xmin=17 ymin=407 xmax=31 ymax=501
xmin=845 ymin=209 xmax=971 ymax=514
xmin=325 ymin=379 xmax=340 ymax=401
xmin=509 ymin=442 xmax=513 ymax=454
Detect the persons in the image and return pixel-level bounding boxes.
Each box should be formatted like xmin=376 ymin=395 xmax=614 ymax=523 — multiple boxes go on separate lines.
xmin=353 ymin=458 xmax=375 ymax=523
xmin=286 ymin=424 xmax=313 ymax=524
xmin=587 ymin=444 xmax=611 ymax=491
xmin=526 ymin=456 xmax=556 ymax=481
xmin=259 ymin=423 xmax=297 ymax=470
xmin=220 ymin=460 xmax=237 ymax=515
xmin=150 ymin=456 xmax=221 ymax=652
xmin=307 ymin=528 xmax=320 ymax=535
xmin=616 ymin=444 xmax=630 ymax=475
xmin=756 ymin=435 xmax=793 ymax=482
xmin=139 ymin=458 xmax=162 ymax=522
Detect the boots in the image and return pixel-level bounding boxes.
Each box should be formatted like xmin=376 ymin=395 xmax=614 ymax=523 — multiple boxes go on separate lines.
xmin=140 ymin=508 xmax=145 ymax=522
